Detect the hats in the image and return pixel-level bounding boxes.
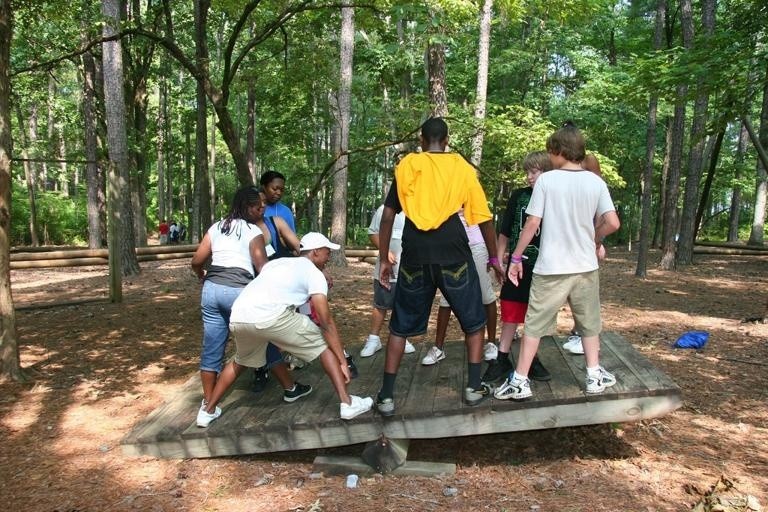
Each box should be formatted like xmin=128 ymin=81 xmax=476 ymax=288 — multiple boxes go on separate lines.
xmin=299 ymin=231 xmax=342 ymax=252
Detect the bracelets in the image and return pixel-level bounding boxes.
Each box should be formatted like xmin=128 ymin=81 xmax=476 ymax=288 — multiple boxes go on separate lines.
xmin=487 ymin=258 xmax=498 ymax=264
xmin=511 ymin=254 xmax=522 ymax=263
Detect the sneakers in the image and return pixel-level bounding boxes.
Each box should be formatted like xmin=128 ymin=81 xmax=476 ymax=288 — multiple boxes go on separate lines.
xmin=404 ymin=339 xmax=416 ymax=353
xmin=421 ymin=346 xmax=446 ymax=365
xmin=374 ymin=390 xmax=395 ymax=417
xmin=569 ymin=336 xmax=584 ymax=355
xmin=465 ymin=343 xmax=551 ymax=405
xmin=360 ymin=338 xmax=382 ymax=357
xmin=252 ymin=351 xmax=373 ymax=422
xmin=196 ymin=405 xmax=222 ymax=428
xmin=562 ymin=336 xmax=579 ymax=350
xmin=586 ymin=367 xmax=616 ymax=394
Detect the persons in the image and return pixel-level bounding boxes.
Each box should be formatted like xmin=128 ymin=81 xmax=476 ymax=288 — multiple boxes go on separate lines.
xmin=190 ymin=186 xmax=312 ymax=405
xmin=260 ymin=170 xmax=305 ymax=371
xmin=251 ymin=185 xmax=358 ymax=392
xmin=562 ymin=153 xmax=602 ymax=354
xmin=158 ymin=220 xmax=186 ymax=245
xmin=372 ymin=118 xmax=507 ymax=418
xmin=197 ymin=231 xmax=373 ymax=429
xmin=481 ymin=150 xmax=554 ymax=384
xmin=360 ymin=204 xmax=415 ymax=358
xmin=421 ymin=208 xmax=498 ymax=365
xmin=494 ymin=127 xmax=620 ymax=399
